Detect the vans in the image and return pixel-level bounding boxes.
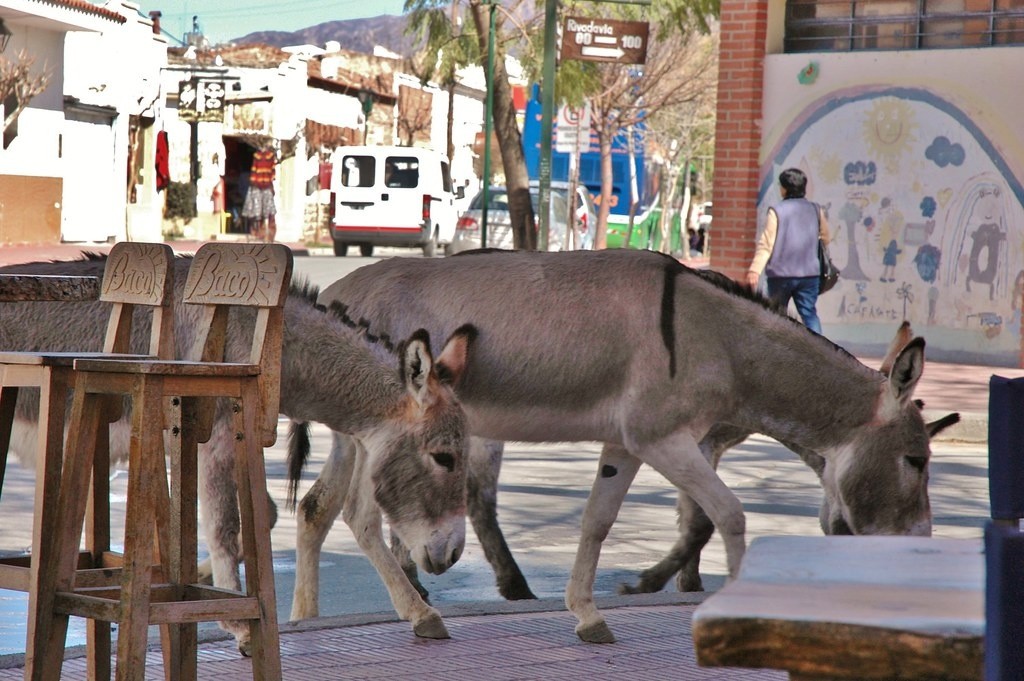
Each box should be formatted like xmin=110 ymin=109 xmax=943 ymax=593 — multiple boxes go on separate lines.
xmin=327 ymin=144 xmax=475 ymax=260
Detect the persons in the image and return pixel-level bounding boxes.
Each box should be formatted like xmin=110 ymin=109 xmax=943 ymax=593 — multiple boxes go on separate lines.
xmin=746 ymin=168 xmax=832 ymax=336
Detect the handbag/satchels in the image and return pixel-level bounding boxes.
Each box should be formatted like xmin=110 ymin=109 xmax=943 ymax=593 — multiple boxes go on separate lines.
xmin=812 ymin=202 xmax=840 ymax=296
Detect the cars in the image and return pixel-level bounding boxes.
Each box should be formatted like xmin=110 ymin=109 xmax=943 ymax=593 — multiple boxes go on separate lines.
xmin=449 ymin=185 xmax=584 ymax=253
xmin=529 ymin=178 xmax=598 ymax=250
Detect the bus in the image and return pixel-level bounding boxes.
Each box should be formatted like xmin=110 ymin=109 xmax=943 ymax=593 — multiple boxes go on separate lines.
xmin=519 ymin=70 xmax=699 ymax=254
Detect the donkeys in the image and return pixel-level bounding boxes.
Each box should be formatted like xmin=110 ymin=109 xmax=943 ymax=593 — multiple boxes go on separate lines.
xmin=1 ymin=249 xmax=477 ymax=660
xmin=286 ymin=246 xmax=931 ymax=606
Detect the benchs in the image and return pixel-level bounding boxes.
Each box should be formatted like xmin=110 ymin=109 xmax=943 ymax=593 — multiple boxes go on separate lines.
xmin=690 ymin=534 xmax=990 ymax=681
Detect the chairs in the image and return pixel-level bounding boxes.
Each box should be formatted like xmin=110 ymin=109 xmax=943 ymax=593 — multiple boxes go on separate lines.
xmin=0 ymin=243 xmax=176 ymax=681
xmin=38 ymin=243 xmax=294 ymax=681
